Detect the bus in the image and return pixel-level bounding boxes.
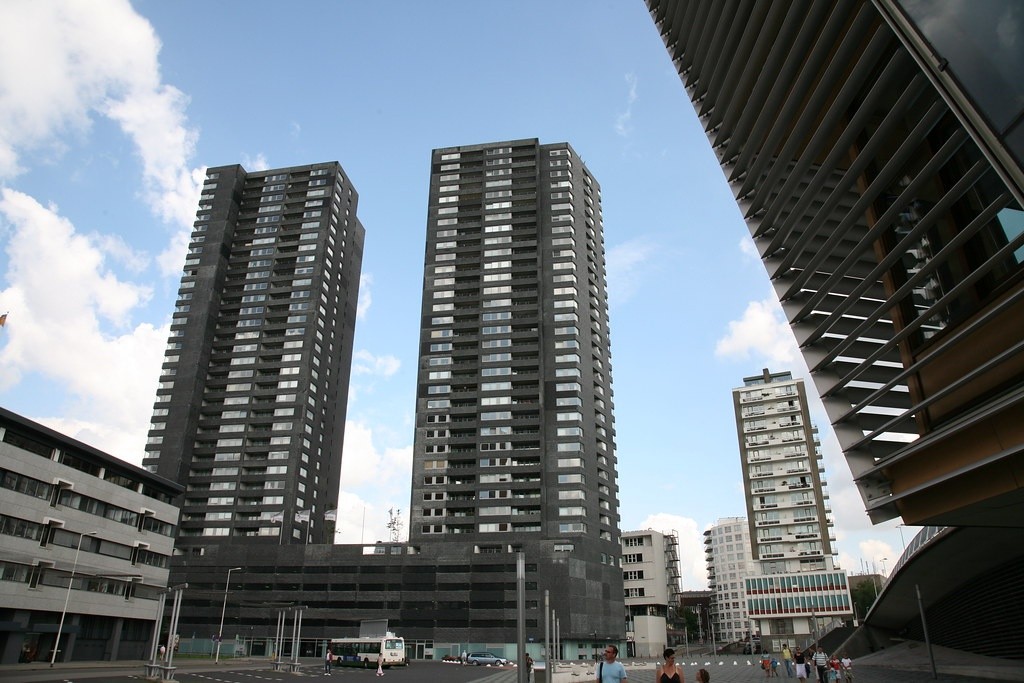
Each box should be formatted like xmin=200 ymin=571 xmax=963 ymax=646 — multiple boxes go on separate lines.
xmin=329 ymin=633 xmax=406 ymax=669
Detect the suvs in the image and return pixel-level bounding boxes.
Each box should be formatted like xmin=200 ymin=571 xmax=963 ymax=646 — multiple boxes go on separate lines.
xmin=467 ymin=652 xmax=507 ymax=666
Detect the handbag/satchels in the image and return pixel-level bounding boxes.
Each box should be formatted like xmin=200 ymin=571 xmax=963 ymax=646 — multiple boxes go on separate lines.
xmin=761 ymin=663 xmax=765 ymax=669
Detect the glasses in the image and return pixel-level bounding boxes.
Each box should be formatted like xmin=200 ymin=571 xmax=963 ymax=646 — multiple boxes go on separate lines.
xmin=605 ymin=650 xmax=614 ymax=653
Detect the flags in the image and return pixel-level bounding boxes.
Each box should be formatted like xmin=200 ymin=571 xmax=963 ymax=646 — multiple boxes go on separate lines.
xmin=0 ymin=314 xmax=7 ymax=327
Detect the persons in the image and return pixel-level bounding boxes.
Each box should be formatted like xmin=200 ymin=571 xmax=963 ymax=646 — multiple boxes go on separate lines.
xmin=596 ymin=645 xmax=629 ymax=683
xmin=159 ymin=644 xmax=165 ymax=660
xmin=526 ymin=653 xmax=533 ymax=683
xmin=462 ymin=650 xmax=467 ymax=666
xmin=324 ymin=649 xmax=333 ymax=675
xmin=794 ymin=647 xmax=807 ymax=683
xmin=376 ymin=653 xmax=385 ymax=676
xmin=770 ymin=658 xmax=778 ymax=677
xmin=781 ymin=644 xmax=794 ymax=678
xmin=808 ymin=646 xmax=855 ymax=683
xmin=656 ymin=649 xmax=685 ymax=683
xmin=761 ymin=649 xmax=771 ymax=677
xmin=696 ymin=669 xmax=710 ymax=683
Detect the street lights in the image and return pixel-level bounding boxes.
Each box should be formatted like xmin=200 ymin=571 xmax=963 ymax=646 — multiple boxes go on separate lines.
xmin=791 ymin=585 xmax=818 ymax=655
xmin=669 ymin=607 xmax=688 ymax=658
xmin=50 ymin=531 xmax=98 ymax=666
xmin=215 ymin=567 xmax=243 ymax=664
xmin=878 ymin=557 xmax=889 ymax=582
xmin=895 ymin=523 xmax=906 ymax=551
xmin=588 ymin=630 xmax=598 ymax=664
xmin=697 ymin=603 xmax=702 ymax=640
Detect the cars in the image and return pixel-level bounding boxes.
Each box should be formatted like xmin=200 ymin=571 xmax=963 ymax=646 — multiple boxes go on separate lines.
xmin=743 ymin=637 xmax=762 ymax=655
